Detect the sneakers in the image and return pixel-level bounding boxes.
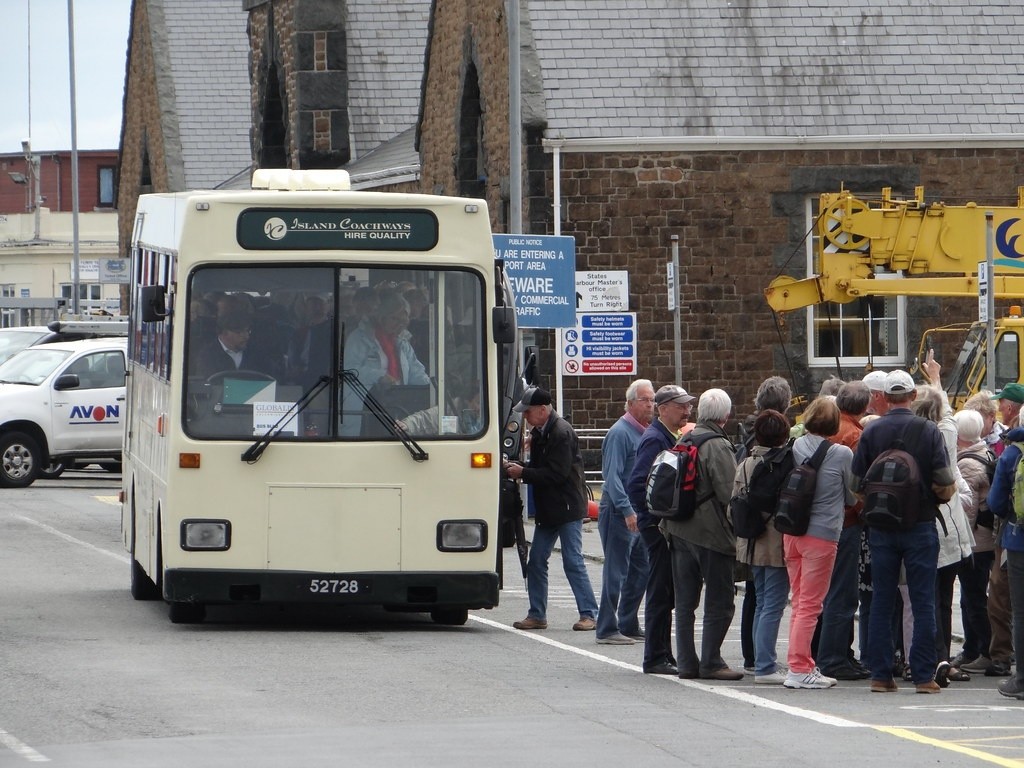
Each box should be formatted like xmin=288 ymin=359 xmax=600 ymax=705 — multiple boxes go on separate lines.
xmin=513 ymin=617 xmax=548 ymax=629
xmin=680 ymin=669 xmax=698 ymax=678
xmin=625 ymin=630 xmax=646 ymax=640
xmin=573 ymin=617 xmax=596 ymax=630
xmin=700 ymin=666 xmax=744 ymax=680
xmin=644 ymin=658 xmax=678 ymax=674
xmin=596 ymin=632 xmax=636 ymax=645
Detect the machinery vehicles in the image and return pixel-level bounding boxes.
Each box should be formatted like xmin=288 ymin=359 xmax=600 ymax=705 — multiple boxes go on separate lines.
xmin=765 ymin=181 xmax=1024 ymax=425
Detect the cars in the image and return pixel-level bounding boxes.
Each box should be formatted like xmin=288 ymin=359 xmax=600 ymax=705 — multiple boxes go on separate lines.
xmin=0 ymin=326 xmax=50 ymax=371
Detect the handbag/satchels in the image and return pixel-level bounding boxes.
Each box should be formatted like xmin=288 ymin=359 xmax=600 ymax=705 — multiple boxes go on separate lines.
xmin=862 ymin=440 xmax=921 ymax=528
xmin=774 ymin=457 xmax=816 ymax=536
xmin=749 ymin=444 xmax=792 ymax=512
xmin=502 ymin=478 xmax=516 ymax=548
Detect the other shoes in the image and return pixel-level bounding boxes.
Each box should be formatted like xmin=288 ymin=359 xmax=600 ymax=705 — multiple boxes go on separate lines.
xmin=742 ymin=650 xmax=1024 ymax=701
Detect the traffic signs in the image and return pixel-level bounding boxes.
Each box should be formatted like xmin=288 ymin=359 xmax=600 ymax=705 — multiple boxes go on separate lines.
xmin=575 ymin=270 xmax=629 ymax=313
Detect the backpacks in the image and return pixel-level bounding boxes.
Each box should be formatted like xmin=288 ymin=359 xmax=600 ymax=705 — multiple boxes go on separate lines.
xmin=1011 ymin=440 xmax=1024 ymax=525
xmin=725 ymin=459 xmax=774 ymax=538
xmin=958 ymin=451 xmax=1000 ymax=529
xmin=646 ymin=430 xmax=728 ymax=521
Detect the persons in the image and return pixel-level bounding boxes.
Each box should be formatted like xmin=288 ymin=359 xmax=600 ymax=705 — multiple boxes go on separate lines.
xmin=187 ymin=279 xmax=483 ymax=436
xmin=658 ymin=388 xmax=745 ymax=680
xmin=727 ymin=347 xmax=1024 ymax=702
xmin=502 ymin=387 xmax=599 ymax=632
xmin=627 ymin=385 xmax=697 ymax=675
xmin=595 ymin=378 xmax=656 ymax=645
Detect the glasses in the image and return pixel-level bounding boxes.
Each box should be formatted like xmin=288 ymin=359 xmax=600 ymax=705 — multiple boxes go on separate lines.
xmin=229 ymin=326 xmax=253 ymax=334
xmin=664 ymin=403 xmax=693 ymax=411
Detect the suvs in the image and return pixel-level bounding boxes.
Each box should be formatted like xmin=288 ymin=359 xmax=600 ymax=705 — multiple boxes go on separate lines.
xmin=0 ymin=338 xmax=130 ymax=488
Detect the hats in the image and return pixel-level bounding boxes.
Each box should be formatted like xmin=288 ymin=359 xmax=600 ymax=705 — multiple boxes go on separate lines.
xmin=987 ymin=383 xmax=1024 ymax=403
xmin=655 ymin=385 xmax=698 ymax=407
xmin=512 ymin=387 xmax=551 ymax=412
xmin=863 ymin=371 xmax=887 ymax=391
xmin=884 ymin=370 xmax=915 ymax=394
xmin=303 ymin=292 xmax=330 ymax=303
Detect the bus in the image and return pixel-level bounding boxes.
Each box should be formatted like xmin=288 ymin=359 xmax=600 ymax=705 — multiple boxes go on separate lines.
xmin=121 ymin=168 xmax=539 ymax=626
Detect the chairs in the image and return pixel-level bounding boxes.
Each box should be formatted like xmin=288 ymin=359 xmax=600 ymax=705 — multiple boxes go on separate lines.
xmin=68 ymin=355 xmax=124 ymax=388
xmin=208 ymin=370 xmax=278 ymax=387
